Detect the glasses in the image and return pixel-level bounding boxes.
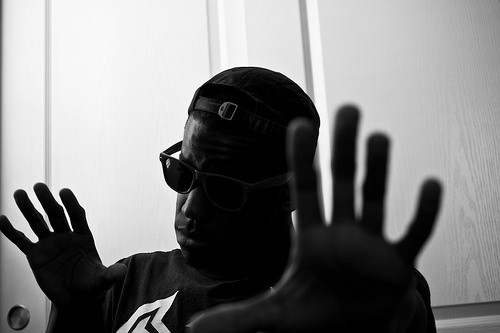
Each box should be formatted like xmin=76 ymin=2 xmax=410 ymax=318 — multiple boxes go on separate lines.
xmin=160 ymin=141 xmax=297 ymax=209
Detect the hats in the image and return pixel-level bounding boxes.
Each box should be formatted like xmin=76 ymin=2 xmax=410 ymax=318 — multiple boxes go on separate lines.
xmin=186 ymin=66 xmax=322 ymax=165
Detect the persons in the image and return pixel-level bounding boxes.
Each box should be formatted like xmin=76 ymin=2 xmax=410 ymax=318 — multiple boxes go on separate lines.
xmin=0 ymin=65 xmax=443 ymax=333
